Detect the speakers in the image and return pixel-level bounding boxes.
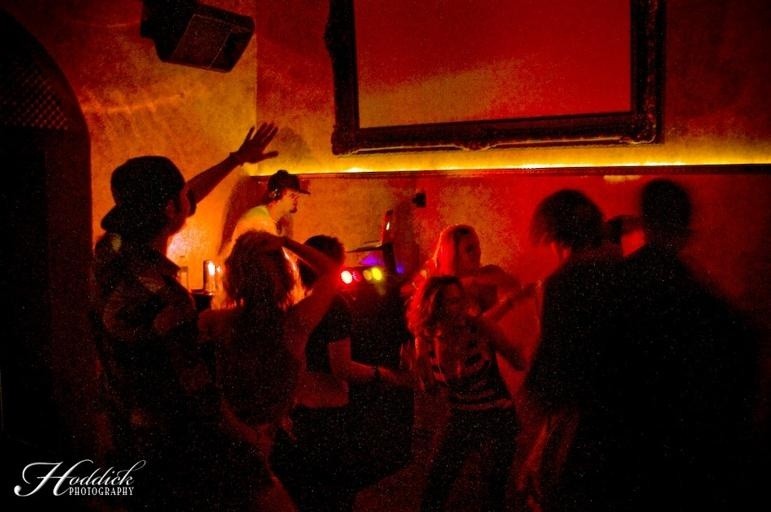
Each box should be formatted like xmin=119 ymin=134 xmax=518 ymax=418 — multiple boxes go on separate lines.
xmin=154 ymin=0 xmax=254 ymax=72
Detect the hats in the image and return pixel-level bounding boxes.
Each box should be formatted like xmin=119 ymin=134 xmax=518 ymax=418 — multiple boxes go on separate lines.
xmin=101 ymin=155 xmax=185 ymax=244
xmin=268 ymin=170 xmax=312 ymax=195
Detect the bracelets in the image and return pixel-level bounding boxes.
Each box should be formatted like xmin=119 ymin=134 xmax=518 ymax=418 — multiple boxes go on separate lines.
xmin=229 ymin=151 xmax=245 ymax=167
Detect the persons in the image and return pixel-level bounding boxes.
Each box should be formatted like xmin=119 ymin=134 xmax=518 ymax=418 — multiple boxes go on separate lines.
xmin=194 ymin=170 xmax=770 ymax=512
xmin=101 ymin=120 xmax=278 ymax=510
